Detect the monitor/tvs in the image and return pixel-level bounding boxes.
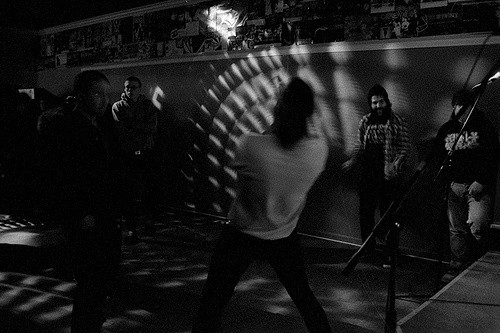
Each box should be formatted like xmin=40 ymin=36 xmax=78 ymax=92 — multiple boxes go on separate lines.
xmin=18 ymin=88 xmax=35 ymax=100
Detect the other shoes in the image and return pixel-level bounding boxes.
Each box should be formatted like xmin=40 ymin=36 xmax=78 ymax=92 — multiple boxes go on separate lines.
xmin=383 ymin=255 xmax=392 ymax=268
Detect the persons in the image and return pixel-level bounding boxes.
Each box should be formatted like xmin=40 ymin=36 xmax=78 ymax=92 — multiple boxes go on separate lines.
xmin=339 ymin=84 xmax=409 ymax=269
xmin=0 ymin=76 xmax=164 ymax=224
xmin=36 ymin=69 xmax=125 ymax=333
xmin=190 ymin=78 xmax=333 ymax=333
xmin=421 ymin=89 xmax=498 ymax=282
